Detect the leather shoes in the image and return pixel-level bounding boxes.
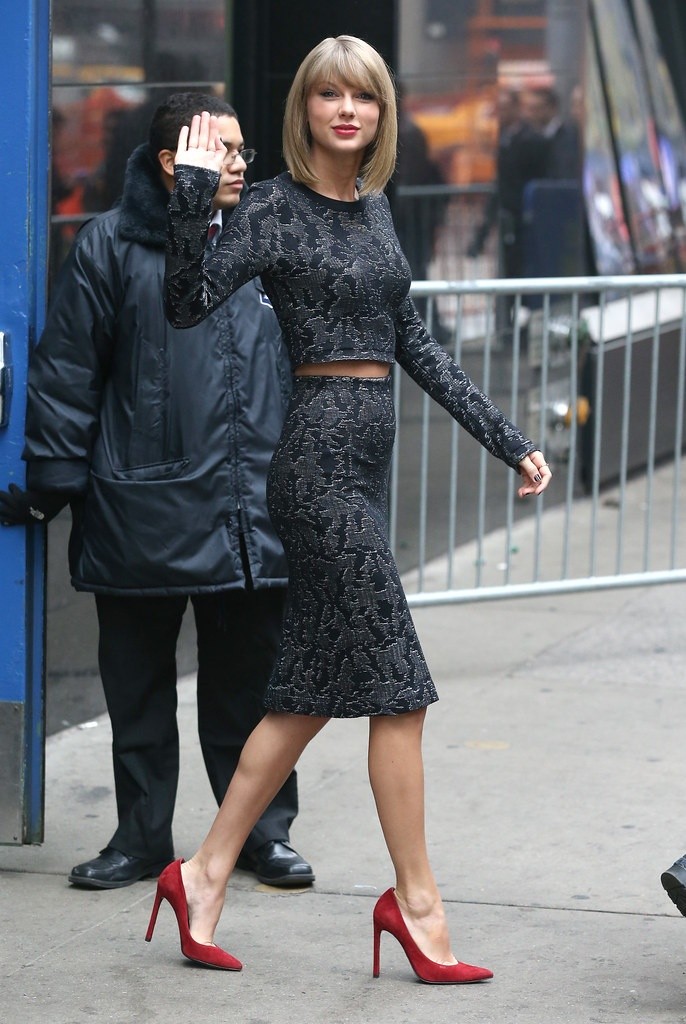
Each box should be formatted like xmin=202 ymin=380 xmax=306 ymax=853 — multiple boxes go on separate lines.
xmin=68 ymin=847 xmax=175 ymax=889
xmin=235 ymin=841 xmax=316 ymax=886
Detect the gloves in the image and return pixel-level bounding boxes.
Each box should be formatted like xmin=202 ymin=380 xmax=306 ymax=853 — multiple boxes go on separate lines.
xmin=0 ymin=483 xmax=69 ymax=527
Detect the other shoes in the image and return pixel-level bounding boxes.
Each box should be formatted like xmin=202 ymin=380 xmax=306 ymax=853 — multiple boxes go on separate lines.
xmin=661 ymin=853 xmax=686 ymax=918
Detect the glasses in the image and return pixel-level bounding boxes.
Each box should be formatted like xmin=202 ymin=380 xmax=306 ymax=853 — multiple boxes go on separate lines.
xmin=223 ymin=147 xmax=258 ymax=164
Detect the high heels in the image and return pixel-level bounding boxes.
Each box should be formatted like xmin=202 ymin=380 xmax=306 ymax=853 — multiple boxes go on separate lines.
xmin=373 ymin=887 xmax=493 ymax=985
xmin=145 ymin=857 xmax=243 ymax=971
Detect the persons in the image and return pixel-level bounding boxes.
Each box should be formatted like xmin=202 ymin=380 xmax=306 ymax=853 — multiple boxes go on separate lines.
xmin=139 ymin=30 xmax=555 ymax=985
xmin=45 ymin=100 xmax=136 ymax=288
xmin=19 ymin=85 xmax=324 ymax=895
xmin=381 ymin=106 xmax=460 ymax=344
xmin=467 ymin=85 xmax=591 ymax=354
